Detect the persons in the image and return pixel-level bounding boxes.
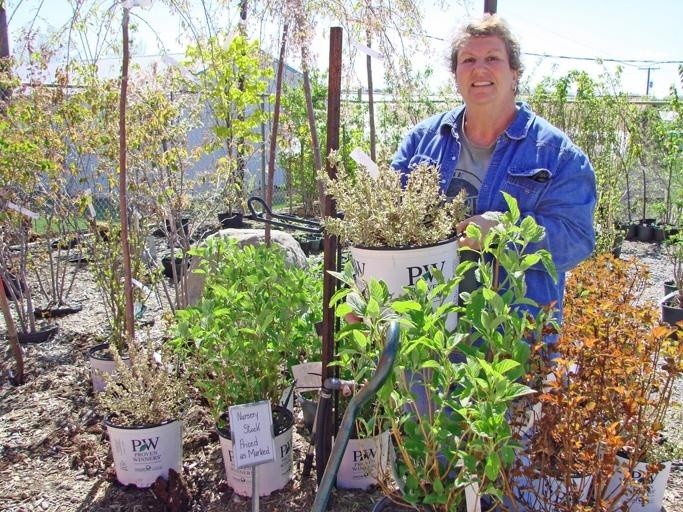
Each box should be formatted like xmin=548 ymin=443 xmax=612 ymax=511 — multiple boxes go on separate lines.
xmin=386 ymin=14 xmax=598 ymax=498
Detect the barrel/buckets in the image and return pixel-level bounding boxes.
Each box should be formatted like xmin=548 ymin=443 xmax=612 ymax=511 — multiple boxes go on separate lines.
xmin=88 ymin=342 xmax=135 ymax=398
xmin=600 ymin=444 xmax=672 ymax=511
xmin=328 ymin=426 xmax=392 ymax=492
xmin=102 ymin=410 xmax=186 ymax=486
xmin=215 ymin=401 xmax=291 ymax=494
xmin=272 ymin=371 xmax=294 ymax=411
xmin=522 ymin=452 xmax=594 ymax=509
xmin=348 ymin=234 xmax=460 ymax=335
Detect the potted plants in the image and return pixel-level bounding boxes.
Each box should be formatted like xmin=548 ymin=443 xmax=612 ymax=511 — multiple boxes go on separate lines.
xmin=370 ymin=187 xmax=561 ymax=512
xmin=323 ymin=263 xmax=395 ymax=491
xmin=164 ymin=237 xmax=324 ymax=497
xmin=97 ymin=324 xmax=188 ymax=491
xmin=511 ymin=347 xmax=594 ymax=511
xmin=75 ymin=224 xmax=160 ymax=395
xmin=298 ymin=346 xmax=323 ymax=425
xmin=315 ymin=149 xmax=467 ymax=336
xmin=598 ymin=317 xmax=682 ymax=511
xmin=585 ymin=96 xmax=682 ymax=338
